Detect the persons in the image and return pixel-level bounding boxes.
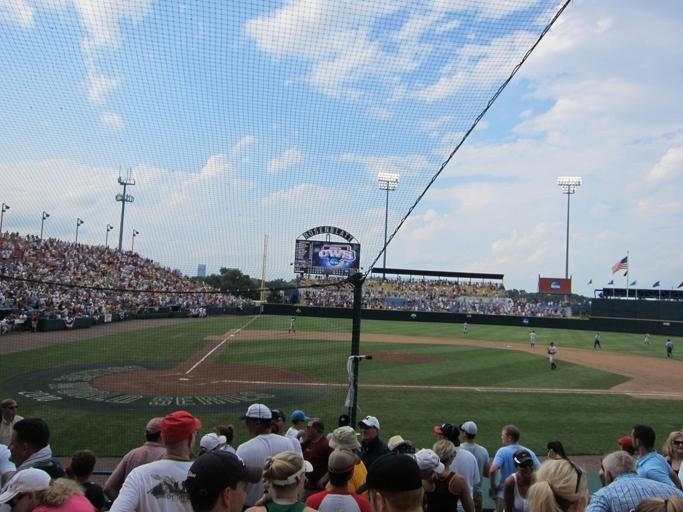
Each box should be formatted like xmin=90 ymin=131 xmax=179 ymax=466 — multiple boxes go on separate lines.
xmin=386 ymin=434 xmax=421 ymax=457
xmin=662 ymin=431 xmax=682 ymax=489
xmin=325 ymin=413 xmax=351 ymax=441
xmin=584 ymin=449 xmax=682 ymax=512
xmin=593 ymin=331 xmax=600 ymax=352
xmin=617 ymin=435 xmax=636 ymax=456
xmin=525 ymin=458 xmax=589 ymax=511
xmin=0 ymin=409 xmax=16 ymax=489
xmin=413 ymin=447 xmax=447 ymax=480
xmin=547 ymin=440 xmax=569 ymax=459
xmin=422 ymin=438 xmax=475 ymax=511
xmin=284 ymin=409 xmax=311 ymax=439
xmin=0 ymin=226 xmax=281 ymax=336
xmin=487 ymin=424 xmax=541 ymax=512
xmin=0 ymin=398 xmax=24 ymax=446
xmin=299 ymin=417 xmax=334 ymax=499
xmin=305 ymin=447 xmax=373 ymax=511
xmin=503 ymin=448 xmax=537 ymax=511
xmin=633 ymin=495 xmax=683 ymax=511
xmin=664 ymin=338 xmax=673 ymax=360
xmin=640 ymin=332 xmax=652 ymax=346
xmin=288 ymin=316 xmax=296 ymax=336
xmin=197 ymin=432 xmax=227 ymax=456
xmin=0 ymin=468 xmax=100 ymax=512
xmin=185 ymin=449 xmax=263 ymax=511
xmin=357 ymin=415 xmax=391 ymax=467
xmin=270 ymin=408 xmax=304 ymax=460
xmin=324 ymin=425 xmax=369 ymax=501
xmin=462 ymin=321 xmax=468 ymax=336
xmin=69 ymin=449 xmax=108 ymax=511
xmin=529 ymin=330 xmax=537 ymax=351
xmin=355 ymin=452 xmax=424 ymax=511
xmin=457 ymin=421 xmax=490 ymax=511
xmin=434 ymin=423 xmax=481 ymax=511
xmin=212 ymin=423 xmax=236 ymax=456
xmin=8 ymin=417 xmax=66 ymax=480
xmin=108 ymin=411 xmax=202 ymax=512
xmin=244 ymin=451 xmax=318 ymax=511
xmin=291 ymin=266 xmax=587 ymax=322
xmin=547 ymin=341 xmax=558 ymax=369
xmin=235 ymin=403 xmax=295 ymax=511
xmin=629 ymin=424 xmax=682 ymax=491
xmin=100 ymin=416 xmax=169 ymax=503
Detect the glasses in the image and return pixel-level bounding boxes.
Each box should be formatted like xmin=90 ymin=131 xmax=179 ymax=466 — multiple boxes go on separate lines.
xmin=673 ymin=440 xmax=683 ymax=445
xmin=570 ymin=459 xmax=582 ymax=494
xmin=519 ymin=460 xmax=533 ymax=468
xmin=8 ymin=405 xmax=19 ymax=410
xmin=359 ymin=424 xmax=371 ymax=430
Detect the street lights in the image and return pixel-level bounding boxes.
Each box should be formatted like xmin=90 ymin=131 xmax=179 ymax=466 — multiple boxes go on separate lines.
xmin=76 ymin=217 xmax=84 ymax=242
xmin=375 ymin=171 xmax=400 ymax=278
xmin=132 ymin=229 xmax=138 ymax=251
xmin=1 ymin=203 xmax=9 ymax=230
xmin=556 ymin=174 xmax=582 ymax=278
xmin=105 ymin=224 xmax=113 ymax=246
xmin=40 ymin=211 xmax=50 ymax=238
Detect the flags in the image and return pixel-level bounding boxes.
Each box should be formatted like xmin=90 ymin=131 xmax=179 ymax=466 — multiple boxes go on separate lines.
xmin=607 ymin=279 xmax=613 ymax=285
xmin=676 ymin=282 xmax=682 ymax=288
xmin=587 ymin=279 xmax=592 ymax=285
xmin=629 ymin=279 xmax=636 ymax=286
xmin=612 ymin=256 xmax=628 ymax=273
xmin=651 ymin=280 xmax=660 ymax=287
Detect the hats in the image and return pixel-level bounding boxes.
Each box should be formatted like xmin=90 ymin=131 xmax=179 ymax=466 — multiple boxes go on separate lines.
xmin=186 ymin=449 xmax=262 ymax=483
xmin=160 ymin=410 xmax=201 ymax=439
xmin=358 ymin=415 xmax=380 ymax=430
xmin=238 ymin=403 xmax=273 ymax=420
xmin=200 ymin=433 xmax=227 ymax=453
xmin=388 ymin=435 xmax=413 ymax=451
xmin=291 ymin=410 xmax=310 ymax=422
xmin=459 ymin=420 xmax=478 ymax=435
xmin=327 ymin=449 xmax=361 ymax=474
xmin=273 ymin=460 xmax=314 ymax=486
xmin=512 ymin=449 xmax=534 ymax=464
xmin=271 ymin=408 xmax=285 ymax=422
xmin=356 ymin=454 xmax=422 ymax=495
xmin=617 ymin=436 xmax=633 ymax=447
xmin=0 ymin=466 xmax=52 ymax=504
xmin=145 ymin=417 xmax=164 ymax=434
xmin=433 ymin=424 xmax=460 ymax=439
xmin=414 ymin=448 xmax=445 ymax=474
xmin=328 ymin=425 xmax=363 ymax=450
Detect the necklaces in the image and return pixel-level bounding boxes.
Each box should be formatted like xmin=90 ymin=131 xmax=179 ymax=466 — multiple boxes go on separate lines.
xmin=276 ymin=496 xmax=295 ymax=499
